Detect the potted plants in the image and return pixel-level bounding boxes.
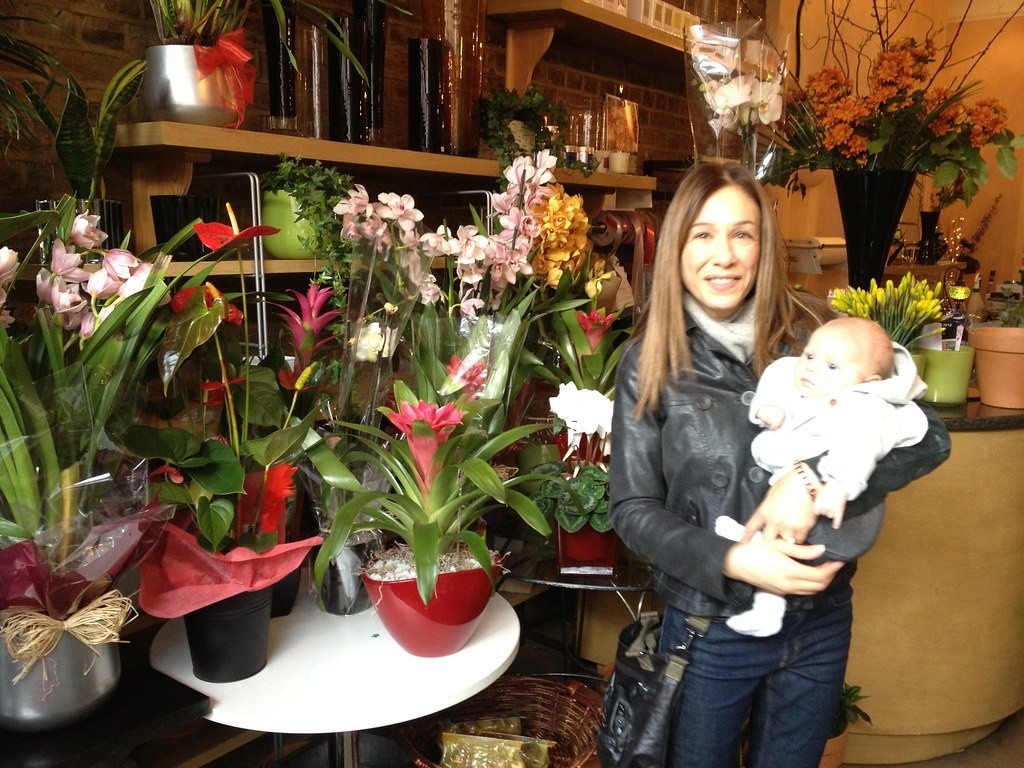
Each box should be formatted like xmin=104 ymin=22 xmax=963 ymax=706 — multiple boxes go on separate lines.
xmin=21 ymin=56 xmax=150 ymax=266
xmin=260 ymin=153 xmax=363 ymax=344
xmin=473 ymin=85 xmax=601 ymax=192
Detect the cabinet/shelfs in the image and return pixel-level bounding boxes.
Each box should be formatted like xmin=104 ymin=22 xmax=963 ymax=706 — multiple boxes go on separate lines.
xmin=482 ymin=0 xmax=778 ymax=100
xmin=0 ymin=122 xmax=657 ymax=768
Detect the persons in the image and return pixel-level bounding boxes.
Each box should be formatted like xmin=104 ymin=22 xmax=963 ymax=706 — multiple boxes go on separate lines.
xmin=715 ymin=316 xmax=929 ymax=637
xmin=605 ymin=160 xmax=952 ymax=768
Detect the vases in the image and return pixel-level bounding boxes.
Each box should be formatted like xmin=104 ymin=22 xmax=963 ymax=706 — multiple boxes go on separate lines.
xmin=358 ymin=555 xmax=501 ymax=657
xmin=920 ymin=210 xmax=940 ymax=264
xmin=322 ymin=15 xmax=351 ymax=144
xmin=556 ymin=515 xmax=618 ymax=579
xmin=311 ymin=540 xmax=383 ymax=618
xmin=970 ymin=323 xmax=1024 ymax=411
xmin=0 ymin=631 xmax=122 ymax=735
xmin=260 ymin=0 xmax=298 ymax=134
xmin=910 ymin=350 xmax=925 ymax=379
xmin=186 ymin=586 xmax=274 ymax=684
xmin=142 ymin=42 xmax=243 ymax=124
xmin=831 ymin=169 xmax=916 ymax=290
xmin=408 ymin=37 xmax=444 ymax=152
xmin=151 ymin=194 xmax=223 ymax=263
xmin=348 ymin=0 xmax=385 ymax=144
xmin=270 ymin=560 xmax=302 ymax=619
xmin=915 ymin=341 xmax=976 ymax=409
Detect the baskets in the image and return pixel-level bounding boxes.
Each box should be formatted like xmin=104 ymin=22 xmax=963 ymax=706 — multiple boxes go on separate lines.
xmin=394 ymin=673 xmax=601 ymax=768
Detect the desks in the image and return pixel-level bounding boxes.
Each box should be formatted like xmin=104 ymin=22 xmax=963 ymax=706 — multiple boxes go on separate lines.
xmin=493 ymin=549 xmax=657 ymax=687
xmin=150 ymin=567 xmax=520 ymax=768
xmin=0 ymin=659 xmax=211 ymax=768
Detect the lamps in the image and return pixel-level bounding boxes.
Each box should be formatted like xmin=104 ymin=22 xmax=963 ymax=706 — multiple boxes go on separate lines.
xmin=944 ymin=266 xmax=971 ymax=311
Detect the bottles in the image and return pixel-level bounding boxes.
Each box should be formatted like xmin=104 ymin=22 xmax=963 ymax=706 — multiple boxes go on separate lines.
xmin=984 ymin=270 xmax=996 ymax=302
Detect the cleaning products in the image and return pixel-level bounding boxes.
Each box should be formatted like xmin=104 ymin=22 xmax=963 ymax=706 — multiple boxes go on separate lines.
xmin=966 ymin=272 xmax=985 ymax=325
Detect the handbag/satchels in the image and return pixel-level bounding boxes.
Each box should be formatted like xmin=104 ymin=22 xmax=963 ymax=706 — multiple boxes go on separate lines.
xmin=598 ymin=617 xmax=691 ymax=768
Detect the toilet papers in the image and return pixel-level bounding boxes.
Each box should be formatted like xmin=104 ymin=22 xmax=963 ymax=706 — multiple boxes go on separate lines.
xmin=805 ymin=235 xmax=848 ymax=268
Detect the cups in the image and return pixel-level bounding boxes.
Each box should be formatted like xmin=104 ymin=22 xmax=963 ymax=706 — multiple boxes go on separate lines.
xmin=557 ymin=97 xmax=605 ymax=170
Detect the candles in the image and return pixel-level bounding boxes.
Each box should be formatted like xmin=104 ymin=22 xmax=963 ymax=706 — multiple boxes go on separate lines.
xmin=609 ymin=152 xmax=631 ymax=174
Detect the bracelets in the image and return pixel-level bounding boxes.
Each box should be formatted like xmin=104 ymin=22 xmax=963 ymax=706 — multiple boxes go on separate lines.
xmin=795 ymin=461 xmax=816 ymax=500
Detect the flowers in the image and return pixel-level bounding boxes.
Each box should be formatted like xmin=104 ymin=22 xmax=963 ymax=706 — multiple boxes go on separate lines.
xmin=773 ymin=0 xmax=1024 ymax=209
xmin=825 ymin=272 xmax=948 ymax=357
xmin=0 ymin=0 xmax=1024 ymax=605
